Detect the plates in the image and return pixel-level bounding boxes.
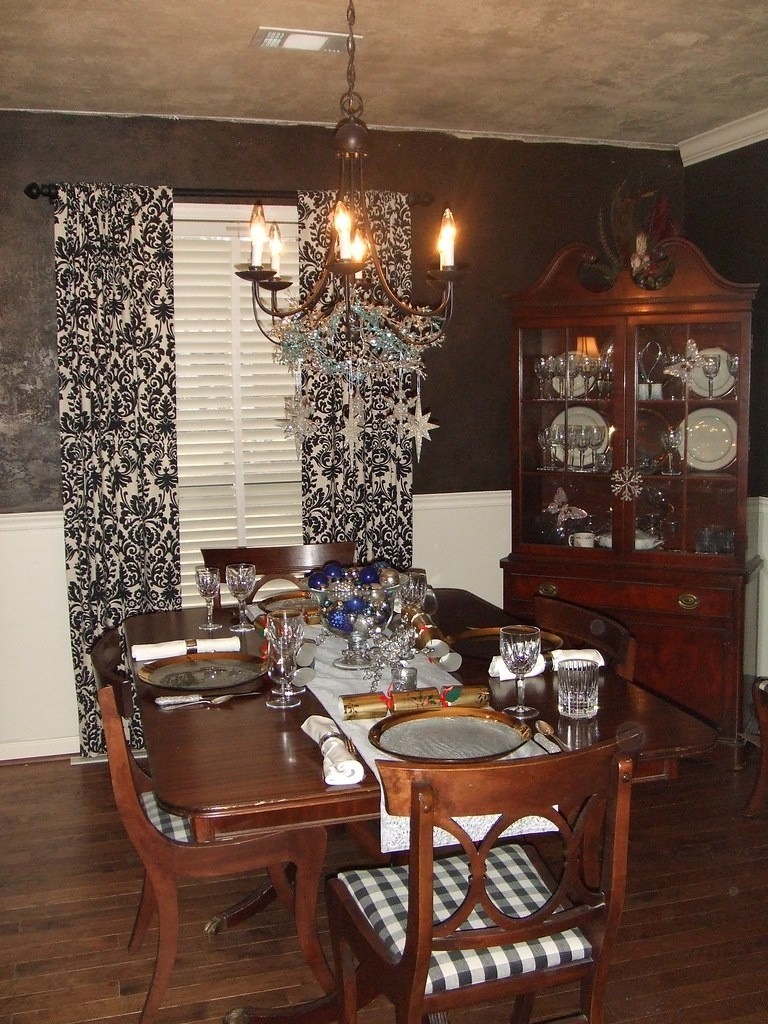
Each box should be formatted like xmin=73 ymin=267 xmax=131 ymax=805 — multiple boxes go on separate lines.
xmin=552 ymin=351 xmax=596 ymax=399
xmin=689 ymin=348 xmax=735 ymax=399
xmin=548 ymin=407 xmax=610 ymax=467
xmin=678 ymin=407 xmax=738 ymax=472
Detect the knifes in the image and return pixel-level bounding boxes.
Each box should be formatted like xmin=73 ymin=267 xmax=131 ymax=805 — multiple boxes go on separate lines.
xmin=154 ymin=691 xmax=261 ymax=705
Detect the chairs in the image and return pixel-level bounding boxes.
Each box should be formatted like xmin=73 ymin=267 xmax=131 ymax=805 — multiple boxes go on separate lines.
xmin=352 ymin=596 xmax=639 ymax=872
xmin=203 ymin=542 xmax=358 ymax=608
xmin=90 ymin=627 xmax=337 ymax=1024
xmin=327 ymin=727 xmax=643 ymax=1023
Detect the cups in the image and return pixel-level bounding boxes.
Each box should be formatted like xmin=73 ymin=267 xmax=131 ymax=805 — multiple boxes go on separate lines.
xmin=557 ymin=659 xmax=601 ymax=719
xmin=401 ymin=572 xmax=426 ymax=608
xmin=569 ymin=532 xmax=595 ymax=547
xmin=594 ymin=446 xmax=612 ymax=474
xmin=391 ymin=667 xmax=418 ymax=691
xmin=694 ymin=523 xmax=736 ymax=556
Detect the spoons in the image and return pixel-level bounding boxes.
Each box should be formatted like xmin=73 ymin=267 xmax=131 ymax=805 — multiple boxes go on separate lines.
xmin=534 ymin=721 xmax=571 ymax=753
xmin=164 ymin=694 xmax=232 ymax=711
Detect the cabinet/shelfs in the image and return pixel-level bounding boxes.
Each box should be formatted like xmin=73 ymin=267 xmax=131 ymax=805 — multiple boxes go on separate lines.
xmin=503 ymin=236 xmax=766 ymax=772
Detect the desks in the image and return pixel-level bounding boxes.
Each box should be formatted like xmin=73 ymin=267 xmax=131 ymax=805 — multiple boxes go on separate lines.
xmin=120 ymin=587 xmax=721 ymax=1024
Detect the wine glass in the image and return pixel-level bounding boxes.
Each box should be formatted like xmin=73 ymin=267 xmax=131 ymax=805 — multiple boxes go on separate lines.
xmin=533 ymin=352 xmax=614 ymax=472
xmin=727 ymin=353 xmax=739 ymax=400
xmin=661 ymin=429 xmax=682 ymax=475
xmin=498 ymin=625 xmax=541 ymax=720
xmin=265 ymin=609 xmax=306 ymax=707
xmin=699 ymin=354 xmax=720 ymax=401
xmin=225 ymin=563 xmax=257 ymax=631
xmin=195 ymin=567 xmax=223 ymax=630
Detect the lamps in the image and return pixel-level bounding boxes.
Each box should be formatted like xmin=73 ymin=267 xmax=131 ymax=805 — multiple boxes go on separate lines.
xmin=235 ymin=0 xmax=471 ymax=469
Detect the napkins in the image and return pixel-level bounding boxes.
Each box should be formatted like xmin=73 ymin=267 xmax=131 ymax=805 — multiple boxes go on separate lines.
xmin=301 ymin=714 xmax=365 ymax=786
xmin=131 ymin=635 xmax=240 ymax=662
xmin=488 ymin=648 xmax=606 ymax=681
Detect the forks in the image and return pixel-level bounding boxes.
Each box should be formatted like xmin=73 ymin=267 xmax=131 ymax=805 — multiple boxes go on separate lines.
xmin=344 ymin=738 xmax=357 ymax=760
